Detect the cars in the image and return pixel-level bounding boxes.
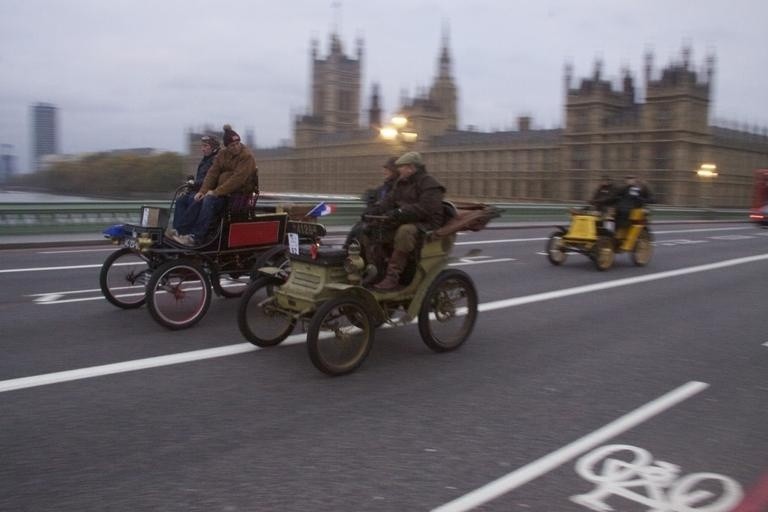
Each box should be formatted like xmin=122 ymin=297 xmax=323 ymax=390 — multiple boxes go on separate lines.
xmin=747 ymin=202 xmax=767 ymax=228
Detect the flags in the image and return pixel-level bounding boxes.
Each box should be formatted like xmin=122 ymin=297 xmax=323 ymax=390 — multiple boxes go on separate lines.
xmin=305 ymin=202 xmax=337 ymax=217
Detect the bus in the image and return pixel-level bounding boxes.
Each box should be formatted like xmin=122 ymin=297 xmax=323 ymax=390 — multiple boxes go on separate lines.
xmin=755 ymin=167 xmax=768 ymax=208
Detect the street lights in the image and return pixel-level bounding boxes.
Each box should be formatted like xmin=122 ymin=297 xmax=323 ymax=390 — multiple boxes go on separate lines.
xmin=691 ymin=161 xmax=719 ymax=207
xmin=377 ymin=114 xmax=419 ymax=157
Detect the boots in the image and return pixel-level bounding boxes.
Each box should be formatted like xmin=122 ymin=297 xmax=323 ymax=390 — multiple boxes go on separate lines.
xmin=373 ymin=252 xmax=409 ymax=291
xmin=364 ymin=243 xmax=383 ymax=284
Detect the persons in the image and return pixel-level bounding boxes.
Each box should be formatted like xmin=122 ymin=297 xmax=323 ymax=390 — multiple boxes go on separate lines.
xmin=164 ymin=136 xmax=219 ymax=237
xmin=581 ymin=174 xmax=655 ymax=237
xmin=342 ymin=158 xmax=400 ymax=255
xmin=170 ymin=125 xmax=257 ymax=246
xmin=361 ymin=151 xmax=446 ymax=291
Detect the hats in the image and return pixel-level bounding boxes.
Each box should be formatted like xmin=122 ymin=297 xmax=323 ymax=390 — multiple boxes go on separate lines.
xmin=382 ymin=156 xmax=400 ymax=172
xmin=222 ymin=123 xmax=241 ymax=147
xmin=201 ymin=134 xmax=221 ymax=152
xmin=395 ymin=150 xmax=422 ymax=167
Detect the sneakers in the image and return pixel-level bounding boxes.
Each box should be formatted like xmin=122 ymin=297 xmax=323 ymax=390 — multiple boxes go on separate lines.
xmin=162 ymin=230 xmax=177 ymax=240
xmin=173 ymin=234 xmax=199 ymax=248
